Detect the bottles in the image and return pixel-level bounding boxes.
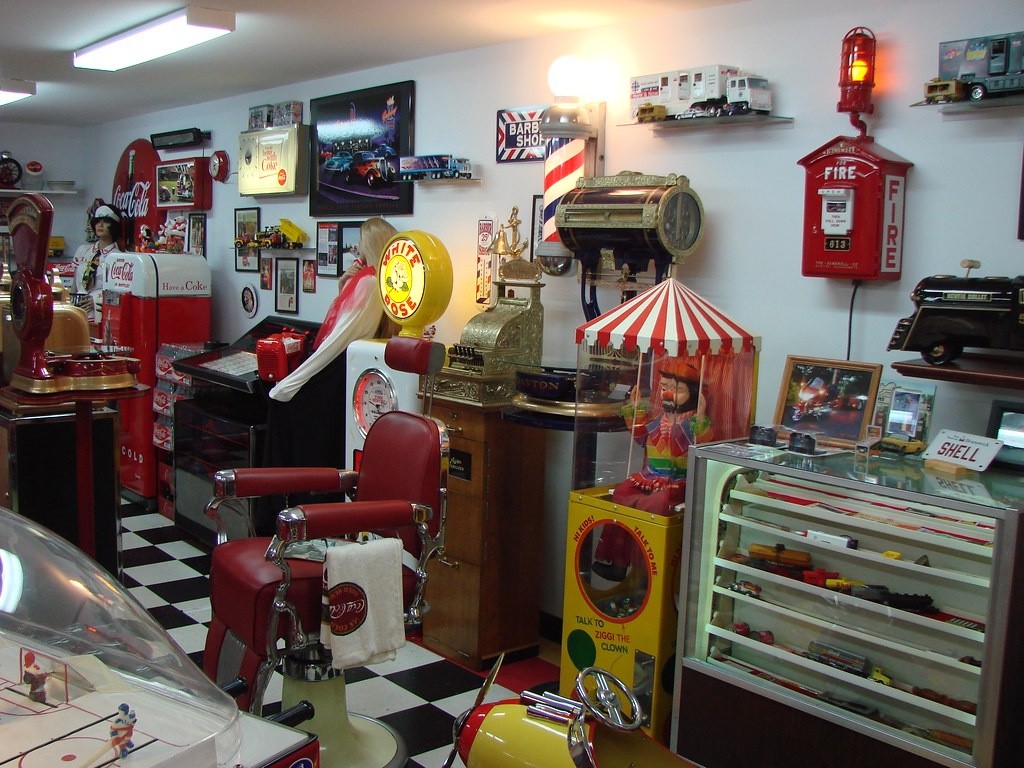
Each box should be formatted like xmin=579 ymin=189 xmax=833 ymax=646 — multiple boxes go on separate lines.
xmin=120 ymin=149 xmax=136 ymax=250
xmin=151 ymin=343 xmax=209 ymax=504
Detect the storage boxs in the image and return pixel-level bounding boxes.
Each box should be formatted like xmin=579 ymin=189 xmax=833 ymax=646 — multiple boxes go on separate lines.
xmin=247 ymin=100 xmax=303 ymax=131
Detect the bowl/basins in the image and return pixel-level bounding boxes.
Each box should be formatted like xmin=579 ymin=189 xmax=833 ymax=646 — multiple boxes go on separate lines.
xmin=47 ymin=180 xmax=75 ymax=191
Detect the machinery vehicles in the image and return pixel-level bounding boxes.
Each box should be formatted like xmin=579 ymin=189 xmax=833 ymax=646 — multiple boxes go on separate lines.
xmin=234 ymin=218 xmax=307 ymax=250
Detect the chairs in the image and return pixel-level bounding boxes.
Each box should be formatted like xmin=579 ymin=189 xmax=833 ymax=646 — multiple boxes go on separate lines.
xmin=198 ymin=410 xmax=448 ymax=714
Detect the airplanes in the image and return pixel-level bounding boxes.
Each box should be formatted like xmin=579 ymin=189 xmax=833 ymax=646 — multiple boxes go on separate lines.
xmin=439 ymin=651 xmax=704 ymax=768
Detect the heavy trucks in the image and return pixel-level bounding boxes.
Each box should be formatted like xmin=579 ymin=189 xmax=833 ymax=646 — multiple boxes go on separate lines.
xmin=399 ymin=154 xmax=472 ymax=180
xmin=630 ymin=64 xmax=773 ymax=118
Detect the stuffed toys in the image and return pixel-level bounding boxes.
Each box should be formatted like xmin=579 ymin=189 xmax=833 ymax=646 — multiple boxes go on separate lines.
xmin=157 ymin=216 xmax=187 ymax=238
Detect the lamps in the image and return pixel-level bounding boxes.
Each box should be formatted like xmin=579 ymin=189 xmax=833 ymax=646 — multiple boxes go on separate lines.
xmin=836 ymin=26 xmax=877 ymax=114
xmin=150 ymin=127 xmax=211 ymax=150
xmin=547 ymin=54 xmax=580 ymax=103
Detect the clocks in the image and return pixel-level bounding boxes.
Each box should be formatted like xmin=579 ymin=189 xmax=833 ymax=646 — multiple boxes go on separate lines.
xmin=236 ymin=135 xmax=259 ymax=190
xmin=208 ymin=150 xmax=230 ymax=181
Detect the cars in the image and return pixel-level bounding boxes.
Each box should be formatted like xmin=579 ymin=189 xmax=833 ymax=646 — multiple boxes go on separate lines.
xmin=880 ymin=433 xmax=926 ymax=456
xmin=886 ymin=274 xmax=1024 ymax=366
xmin=675 ymin=108 xmax=709 ymax=120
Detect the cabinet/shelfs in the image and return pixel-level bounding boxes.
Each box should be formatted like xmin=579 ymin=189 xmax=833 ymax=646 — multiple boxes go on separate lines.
xmin=669 ymin=437 xmax=1024 ymax=768
xmin=238 ymin=124 xmax=310 ymax=198
xmin=414 ymin=391 xmax=547 ymax=673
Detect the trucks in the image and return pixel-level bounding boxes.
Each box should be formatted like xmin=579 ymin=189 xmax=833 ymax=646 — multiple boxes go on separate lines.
xmin=938 ymin=31 xmax=1024 ymax=101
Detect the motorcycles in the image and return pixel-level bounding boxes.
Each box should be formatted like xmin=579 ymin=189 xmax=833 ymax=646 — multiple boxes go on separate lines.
xmin=790 ymin=377 xmax=839 ymax=423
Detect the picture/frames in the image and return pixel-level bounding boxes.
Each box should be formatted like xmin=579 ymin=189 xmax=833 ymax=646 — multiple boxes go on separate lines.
xmin=187 ymin=213 xmax=206 ymax=261
xmin=151 ymin=157 xmax=203 ymax=211
xmin=772 ymin=354 xmax=883 ymax=451
xmin=982 ymin=399 xmax=1024 ymax=478
xmin=336 ymin=221 xmax=367 ymax=277
xmin=275 ymin=257 xmax=300 ymax=315
xmin=234 ymin=207 xmax=261 ymax=274
xmin=239 ymin=283 xmax=258 ymax=318
xmin=530 ymin=195 xmax=544 ymax=263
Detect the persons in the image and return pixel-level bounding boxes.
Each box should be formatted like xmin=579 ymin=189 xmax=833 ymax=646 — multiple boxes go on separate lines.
xmin=68 ymin=204 xmax=123 ymax=324
xmin=592 ymin=362 xmax=718 ymax=582
xmin=269 ymin=217 xmax=401 ymax=403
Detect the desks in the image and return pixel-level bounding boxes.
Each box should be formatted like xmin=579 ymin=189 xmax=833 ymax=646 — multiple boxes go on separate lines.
xmin=502 ymin=408 xmax=627 ymax=585
